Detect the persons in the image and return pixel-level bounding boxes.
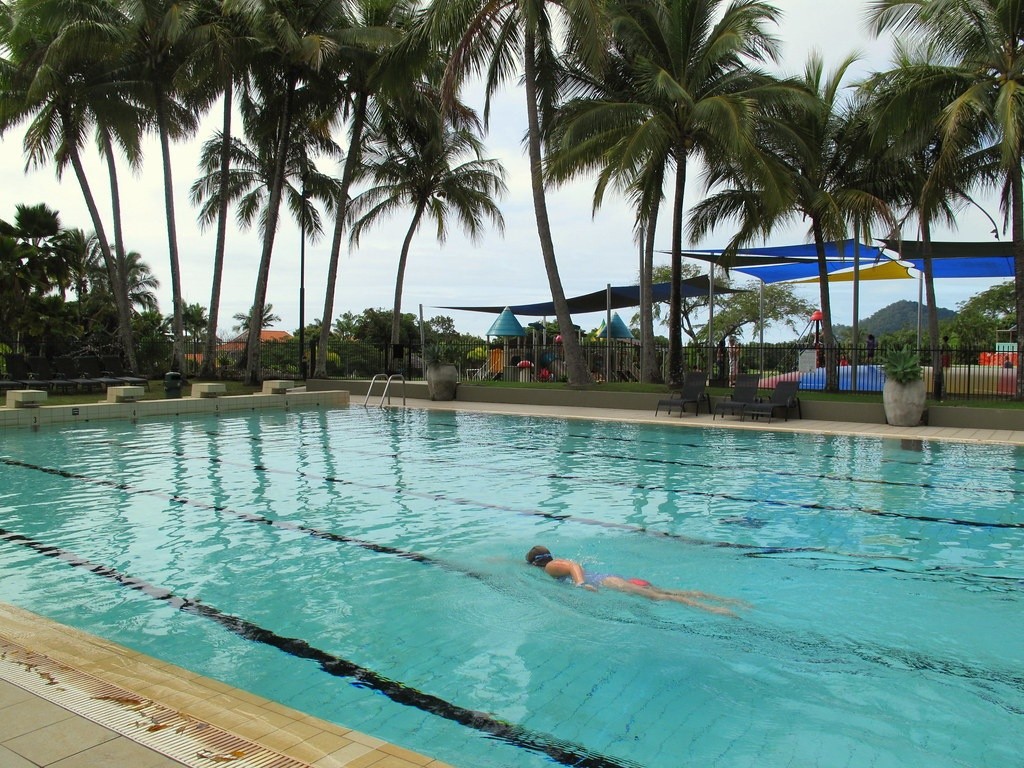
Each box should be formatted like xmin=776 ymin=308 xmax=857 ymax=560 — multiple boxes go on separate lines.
xmin=864 ymin=334 xmax=879 ymax=365
xmin=940 ymin=336 xmax=953 ymax=368
xmin=717 ymin=339 xmax=728 ymax=381
xmin=727 ymin=336 xmax=742 ymax=386
xmin=1002 ymin=354 xmax=1013 ymax=368
xmin=526 ymin=545 xmax=599 ymax=592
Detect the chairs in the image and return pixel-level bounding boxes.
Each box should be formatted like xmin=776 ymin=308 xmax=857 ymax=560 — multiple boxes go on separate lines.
xmin=742 ymin=381 xmax=802 ymax=423
xmin=0 ymin=354 xmax=151 ymax=396
xmin=655 ymin=372 xmax=711 ymax=417
xmin=713 ymin=374 xmax=760 ymax=421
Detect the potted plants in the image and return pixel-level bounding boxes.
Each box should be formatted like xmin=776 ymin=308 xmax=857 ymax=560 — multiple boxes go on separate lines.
xmin=421 ymin=338 xmax=459 ymax=401
xmin=877 ymin=342 xmax=926 ymax=427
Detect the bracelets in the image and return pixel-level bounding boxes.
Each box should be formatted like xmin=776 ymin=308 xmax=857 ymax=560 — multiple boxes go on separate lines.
xmin=578 ymin=582 xmax=588 ymax=588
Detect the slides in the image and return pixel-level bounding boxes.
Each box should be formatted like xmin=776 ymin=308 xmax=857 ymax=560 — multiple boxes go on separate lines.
xmin=612 ymin=369 xmax=640 ymax=382
